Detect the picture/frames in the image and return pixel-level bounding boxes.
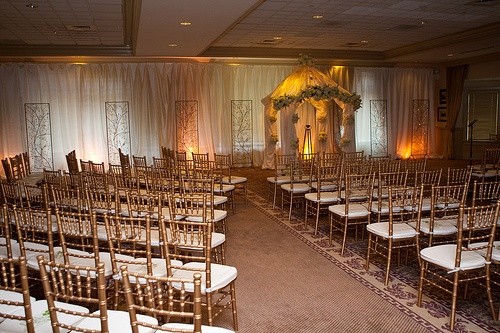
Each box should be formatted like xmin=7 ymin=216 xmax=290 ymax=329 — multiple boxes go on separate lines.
xmin=439 ymin=88 xmax=448 ymax=104
xmin=437 ymin=107 xmax=448 ymax=122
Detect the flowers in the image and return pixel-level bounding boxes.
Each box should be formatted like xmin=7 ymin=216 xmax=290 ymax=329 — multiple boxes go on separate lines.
xmin=267 ymin=84 xmax=363 ymax=149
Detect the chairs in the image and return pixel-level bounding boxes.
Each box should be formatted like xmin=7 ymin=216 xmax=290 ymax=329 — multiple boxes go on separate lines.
xmin=0 ymin=147 xmax=500 ymax=333
